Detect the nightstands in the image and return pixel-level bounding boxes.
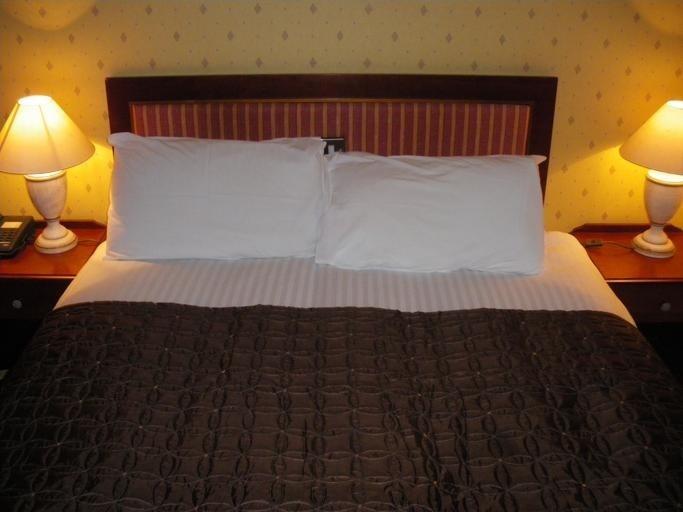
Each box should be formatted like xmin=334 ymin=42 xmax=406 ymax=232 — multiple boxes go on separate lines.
xmin=568 ymin=222 xmax=683 ymax=326
xmin=0 ymin=218 xmax=106 ymax=323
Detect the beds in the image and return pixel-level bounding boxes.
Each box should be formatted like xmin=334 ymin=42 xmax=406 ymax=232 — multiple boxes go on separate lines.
xmin=2 ymin=73 xmax=683 ymax=512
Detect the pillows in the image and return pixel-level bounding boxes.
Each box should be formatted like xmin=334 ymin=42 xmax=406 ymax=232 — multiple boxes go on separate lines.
xmin=315 ymin=146 xmax=549 ymax=278
xmin=107 ymin=130 xmax=328 ymax=264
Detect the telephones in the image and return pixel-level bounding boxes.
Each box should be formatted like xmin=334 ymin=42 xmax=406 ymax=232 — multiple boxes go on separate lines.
xmin=0 ymin=213 xmax=36 ymax=258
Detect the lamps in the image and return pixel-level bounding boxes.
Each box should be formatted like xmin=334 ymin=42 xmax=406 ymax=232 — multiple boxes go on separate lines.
xmin=1 ymin=93 xmax=97 ymax=257
xmin=619 ymin=98 xmax=682 ymax=261
